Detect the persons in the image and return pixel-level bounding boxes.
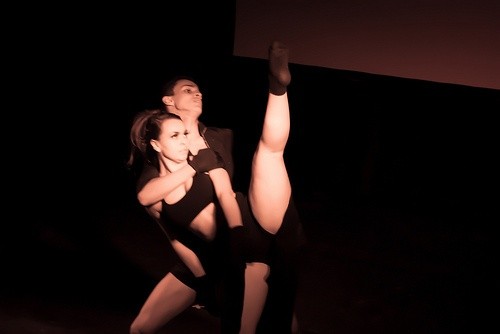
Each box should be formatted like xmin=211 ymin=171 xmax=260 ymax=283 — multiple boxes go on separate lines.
xmin=126 ymin=77 xmax=248 ymax=334
xmin=129 ymin=32 xmax=297 ymax=334
